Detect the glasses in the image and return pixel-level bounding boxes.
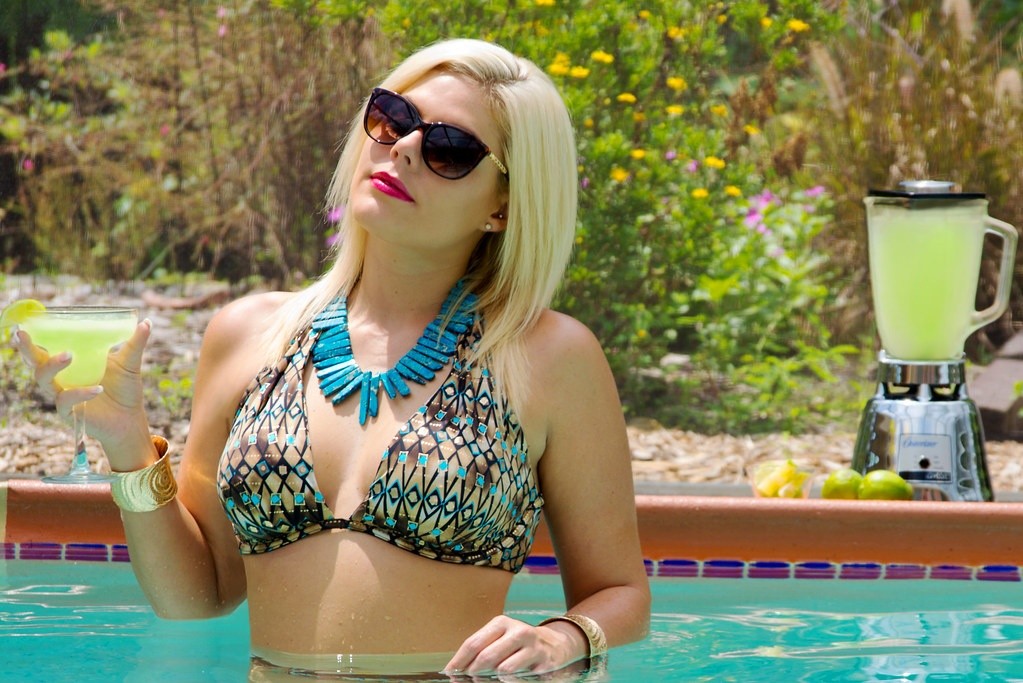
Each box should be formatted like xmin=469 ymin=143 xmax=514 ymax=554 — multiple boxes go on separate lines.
xmin=364 ymin=87 xmax=511 ymax=181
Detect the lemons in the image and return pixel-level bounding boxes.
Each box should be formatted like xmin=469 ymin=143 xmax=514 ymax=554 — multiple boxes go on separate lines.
xmin=754 ymin=459 xmax=916 ymax=501
xmin=0 ymin=299 xmax=46 ymax=329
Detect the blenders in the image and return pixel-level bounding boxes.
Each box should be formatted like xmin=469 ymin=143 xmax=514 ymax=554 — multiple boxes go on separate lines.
xmin=852 ymin=179 xmax=1019 ymax=503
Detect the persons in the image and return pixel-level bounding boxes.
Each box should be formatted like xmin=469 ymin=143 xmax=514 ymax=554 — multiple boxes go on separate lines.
xmin=13 ymin=39 xmax=650 ymax=673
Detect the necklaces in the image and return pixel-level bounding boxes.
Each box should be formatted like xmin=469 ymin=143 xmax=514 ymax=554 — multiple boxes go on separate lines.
xmin=312 ymin=283 xmax=480 ymax=426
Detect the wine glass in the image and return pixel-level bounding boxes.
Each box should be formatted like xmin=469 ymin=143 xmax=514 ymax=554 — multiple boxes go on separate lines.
xmin=13 ymin=305 xmax=138 ymax=483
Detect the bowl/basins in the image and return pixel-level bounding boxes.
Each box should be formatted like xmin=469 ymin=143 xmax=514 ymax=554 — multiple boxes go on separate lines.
xmin=745 ymin=459 xmax=816 ymax=499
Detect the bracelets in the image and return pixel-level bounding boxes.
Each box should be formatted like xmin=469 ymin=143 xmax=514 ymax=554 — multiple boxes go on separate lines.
xmin=109 ymin=434 xmax=178 ymax=513
xmin=538 ymin=614 xmax=606 ymax=658
